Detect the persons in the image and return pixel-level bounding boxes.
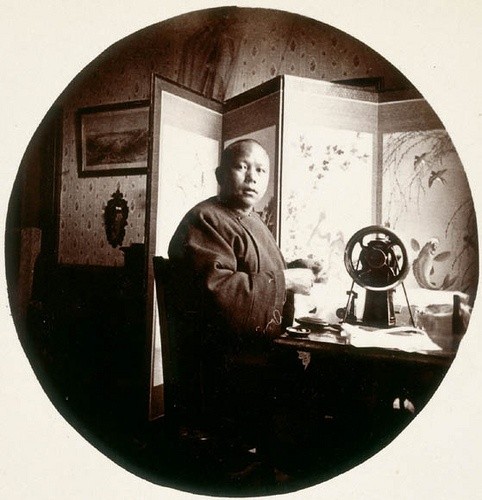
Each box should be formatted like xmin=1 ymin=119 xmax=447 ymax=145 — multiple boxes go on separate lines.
xmin=167 ymin=137 xmax=325 ymax=364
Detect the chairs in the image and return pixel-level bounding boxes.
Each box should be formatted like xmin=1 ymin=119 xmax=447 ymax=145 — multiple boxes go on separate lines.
xmin=153 ymin=257 xmax=200 ymax=417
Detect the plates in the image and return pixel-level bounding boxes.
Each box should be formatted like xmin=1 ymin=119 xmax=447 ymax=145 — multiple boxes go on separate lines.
xmin=286 ymin=326 xmax=311 ymax=337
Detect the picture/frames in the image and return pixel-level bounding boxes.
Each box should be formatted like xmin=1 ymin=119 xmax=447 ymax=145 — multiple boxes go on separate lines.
xmin=75 ymin=100 xmax=151 ymax=177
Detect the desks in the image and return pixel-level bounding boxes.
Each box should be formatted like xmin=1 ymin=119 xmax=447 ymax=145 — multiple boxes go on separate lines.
xmin=271 ymin=313 xmax=452 ymax=368
